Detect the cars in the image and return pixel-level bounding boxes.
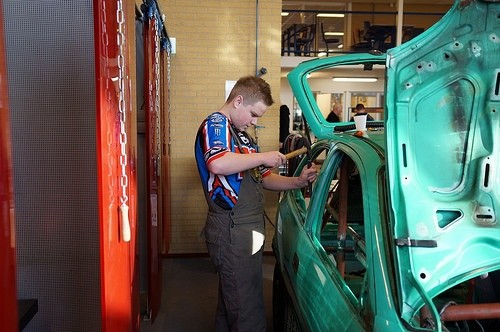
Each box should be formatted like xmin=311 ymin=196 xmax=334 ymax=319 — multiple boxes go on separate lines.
xmin=271 ymin=0 xmax=500 ymax=332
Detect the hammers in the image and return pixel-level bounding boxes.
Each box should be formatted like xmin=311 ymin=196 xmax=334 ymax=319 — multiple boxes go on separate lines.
xmin=285 ymin=138 xmax=312 ymax=161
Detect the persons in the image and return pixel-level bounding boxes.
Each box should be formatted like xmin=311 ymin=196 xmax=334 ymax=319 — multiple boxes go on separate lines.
xmin=349 ymin=104 xmax=374 ymax=122
xmin=279 ymin=101 xmax=290 ymax=177
xmin=194 ymin=75 xmax=317 ymax=332
xmin=326 ymin=103 xmax=343 ymax=123
